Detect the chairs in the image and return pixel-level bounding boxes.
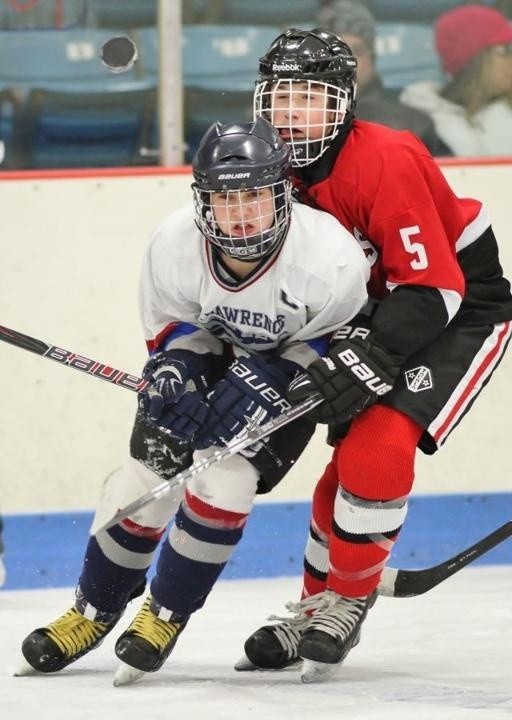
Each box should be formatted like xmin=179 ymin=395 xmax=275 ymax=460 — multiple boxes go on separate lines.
xmin=2 ymin=0 xmax=455 ymax=171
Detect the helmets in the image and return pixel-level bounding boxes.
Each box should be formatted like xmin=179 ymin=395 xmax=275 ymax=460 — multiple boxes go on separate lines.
xmin=253 ymin=27 xmax=358 ymax=170
xmin=191 ymin=117 xmax=294 ymax=262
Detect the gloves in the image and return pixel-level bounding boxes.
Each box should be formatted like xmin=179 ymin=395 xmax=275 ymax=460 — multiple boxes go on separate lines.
xmin=141 ymin=350 xmax=210 ymax=437
xmin=190 ymin=353 xmax=296 ymax=450
xmin=288 ymin=339 xmax=399 ymax=427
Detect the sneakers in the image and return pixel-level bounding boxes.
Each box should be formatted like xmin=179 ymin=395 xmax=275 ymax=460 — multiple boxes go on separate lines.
xmin=242 ymin=603 xmax=308 ymax=672
xmin=297 ymin=587 xmax=380 ymax=664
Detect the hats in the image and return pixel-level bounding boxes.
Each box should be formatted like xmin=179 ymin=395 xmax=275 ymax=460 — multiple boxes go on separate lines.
xmin=315 ymin=0 xmax=375 ymax=43
xmin=437 ymin=5 xmax=512 ymax=75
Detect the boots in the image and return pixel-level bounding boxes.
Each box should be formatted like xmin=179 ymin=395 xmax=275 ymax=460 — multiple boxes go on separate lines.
xmin=113 ymin=584 xmax=192 ymax=673
xmin=22 ymin=575 xmax=150 ymax=675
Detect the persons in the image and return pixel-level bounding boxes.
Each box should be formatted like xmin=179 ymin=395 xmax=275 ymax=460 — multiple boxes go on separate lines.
xmin=311 ymin=0 xmax=457 ymax=160
xmin=398 ymin=3 xmax=512 ymax=159
xmin=244 ymin=23 xmax=511 ymax=669
xmin=22 ymin=121 xmax=376 ymax=673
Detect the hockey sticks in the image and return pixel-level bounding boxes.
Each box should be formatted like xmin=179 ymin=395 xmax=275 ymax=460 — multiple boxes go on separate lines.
xmin=92 ymin=393 xmax=324 ymax=535
xmin=376 ymin=521 xmax=512 ymax=597
